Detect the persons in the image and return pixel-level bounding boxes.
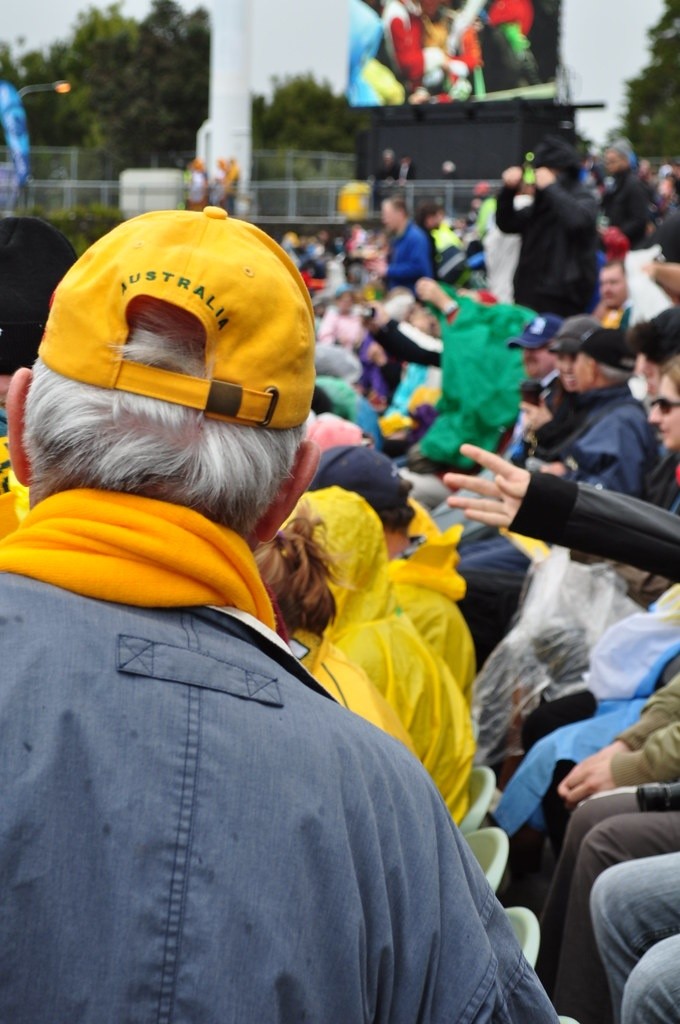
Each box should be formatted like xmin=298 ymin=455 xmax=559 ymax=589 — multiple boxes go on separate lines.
xmin=373 ymin=146 xmax=399 ymax=210
xmin=599 ymin=138 xmax=652 ymax=260
xmin=441 ymin=159 xmax=470 ymax=178
xmin=475 ymin=161 xmax=537 ymax=303
xmin=592 ymin=260 xmax=633 ymax=331
xmin=394 ymin=154 xmax=416 ymax=181
xmin=494 ymin=136 xmax=599 ymax=319
xmin=182 ymin=158 xmax=209 ymax=212
xmin=0 ymin=185 xmax=560 ymax=1024
xmin=579 ymin=144 xmax=614 ymax=207
xmin=441 ymin=314 xmax=679 ymax=1024
xmin=216 ymin=157 xmax=241 ymax=215
xmin=636 ymin=154 xmax=680 ymax=239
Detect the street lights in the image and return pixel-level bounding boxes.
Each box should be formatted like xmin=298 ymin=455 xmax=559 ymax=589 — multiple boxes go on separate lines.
xmin=1 ymin=80 xmax=69 ymax=118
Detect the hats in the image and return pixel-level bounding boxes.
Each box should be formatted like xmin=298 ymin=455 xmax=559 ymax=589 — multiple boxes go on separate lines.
xmin=506 ymin=313 xmax=563 ymax=349
xmin=36 ymin=206 xmax=317 ymax=431
xmin=548 ymin=314 xmax=601 ymax=352
xmin=306 ymin=444 xmax=413 ymax=509
xmin=306 ymin=412 xmax=363 ymax=451
xmin=1 ymin=217 xmax=77 ymax=375
xmin=579 ymin=327 xmax=636 ymax=372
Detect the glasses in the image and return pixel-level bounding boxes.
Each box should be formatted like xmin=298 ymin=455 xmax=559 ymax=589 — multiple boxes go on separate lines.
xmin=649 ymin=398 xmax=680 ymax=413
